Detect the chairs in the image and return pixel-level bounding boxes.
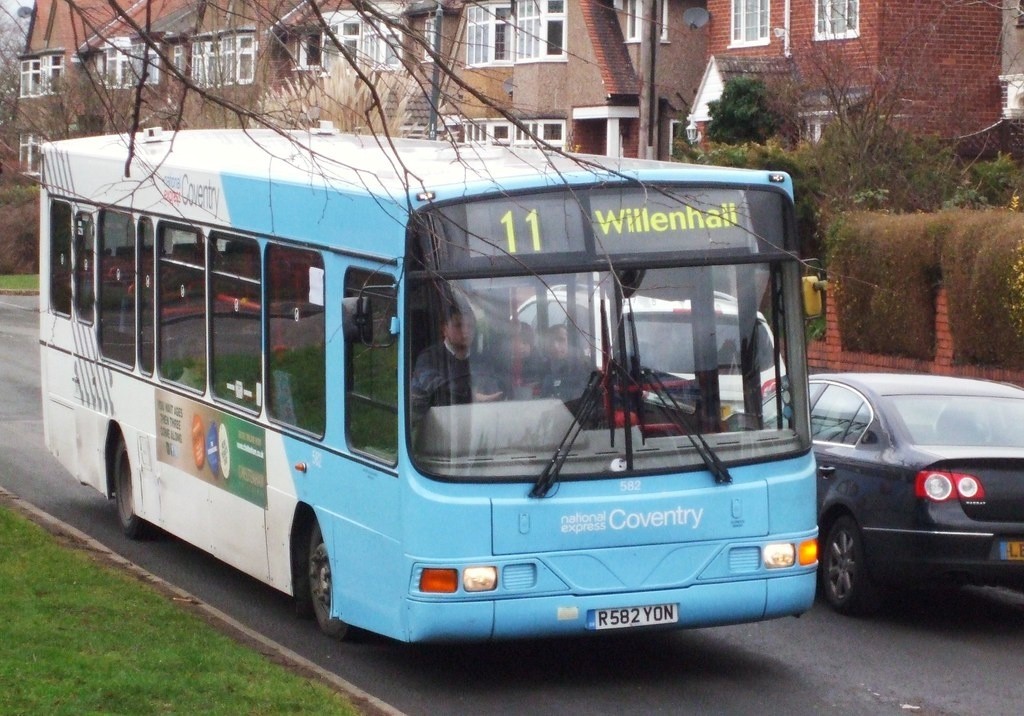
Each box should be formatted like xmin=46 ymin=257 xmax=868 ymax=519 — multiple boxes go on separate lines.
xmin=55 ymin=243 xmax=325 ymax=436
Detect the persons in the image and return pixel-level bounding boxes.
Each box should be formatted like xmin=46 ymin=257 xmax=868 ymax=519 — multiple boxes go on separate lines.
xmin=496 ymin=320 xmax=543 ymax=382
xmin=531 ymin=325 xmax=595 ymax=398
xmin=411 ymin=305 xmax=506 ymax=450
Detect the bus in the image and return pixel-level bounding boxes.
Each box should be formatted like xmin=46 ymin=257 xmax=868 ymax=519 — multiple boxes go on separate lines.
xmin=40 ymin=119 xmax=830 ymax=649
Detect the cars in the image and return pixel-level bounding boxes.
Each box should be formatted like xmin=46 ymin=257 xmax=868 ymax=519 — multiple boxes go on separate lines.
xmin=727 ymin=372 xmax=1024 ymax=615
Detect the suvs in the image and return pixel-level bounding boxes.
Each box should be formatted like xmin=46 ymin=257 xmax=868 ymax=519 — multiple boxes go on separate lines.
xmin=507 ymin=283 xmax=786 ymax=425
